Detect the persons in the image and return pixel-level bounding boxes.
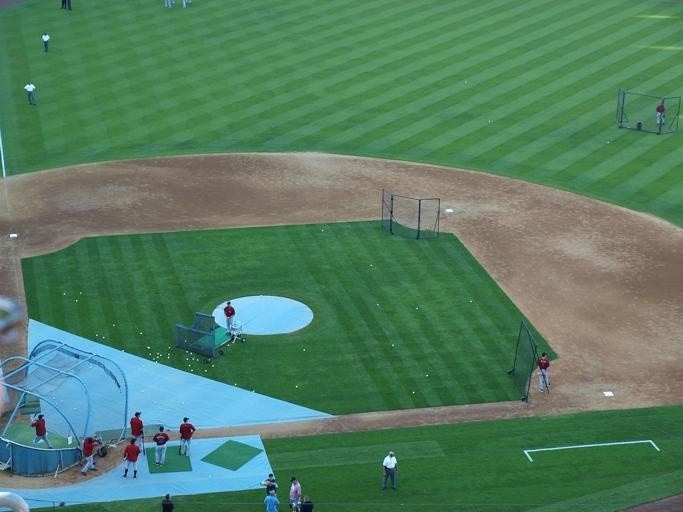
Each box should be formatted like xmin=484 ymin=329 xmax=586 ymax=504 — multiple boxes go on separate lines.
xmin=223 ymin=302 xmax=235 ymax=333
xmin=383 ymin=451 xmax=397 ymax=490
xmin=537 ymin=352 xmax=549 ymax=392
xmin=30 ymin=414 xmax=313 ymax=512
xmin=42 ymin=33 xmax=50 ymax=53
xmin=62 ymin=0 xmax=66 ymax=8
xmin=655 ymin=100 xmax=665 ymax=123
xmin=24 ymin=82 xmax=37 ymax=105
